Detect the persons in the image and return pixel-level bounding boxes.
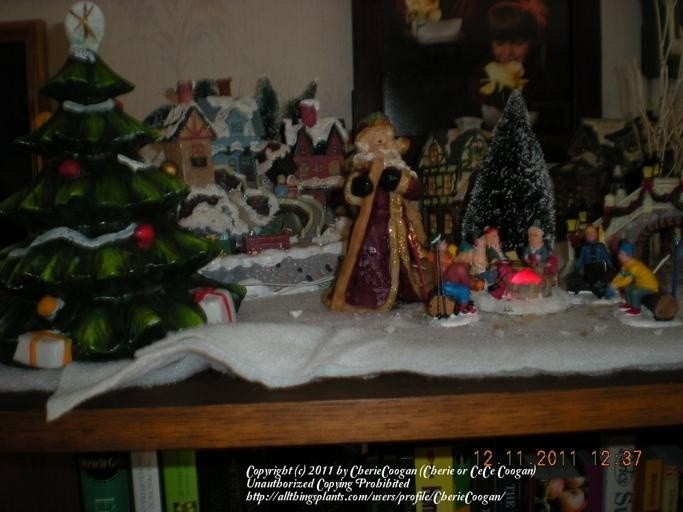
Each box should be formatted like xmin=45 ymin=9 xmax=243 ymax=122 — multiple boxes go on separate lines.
xmin=0 ymin=40 xmax=683 ymax=367
xmin=0 ymin=41 xmax=682 ymax=365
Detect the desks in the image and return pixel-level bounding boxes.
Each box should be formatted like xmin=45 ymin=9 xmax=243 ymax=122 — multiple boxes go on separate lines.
xmin=0 ymin=241 xmax=683 ymax=512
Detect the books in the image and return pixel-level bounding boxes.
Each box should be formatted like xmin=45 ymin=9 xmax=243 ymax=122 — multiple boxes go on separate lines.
xmin=75 ymin=446 xmax=683 ymax=512
